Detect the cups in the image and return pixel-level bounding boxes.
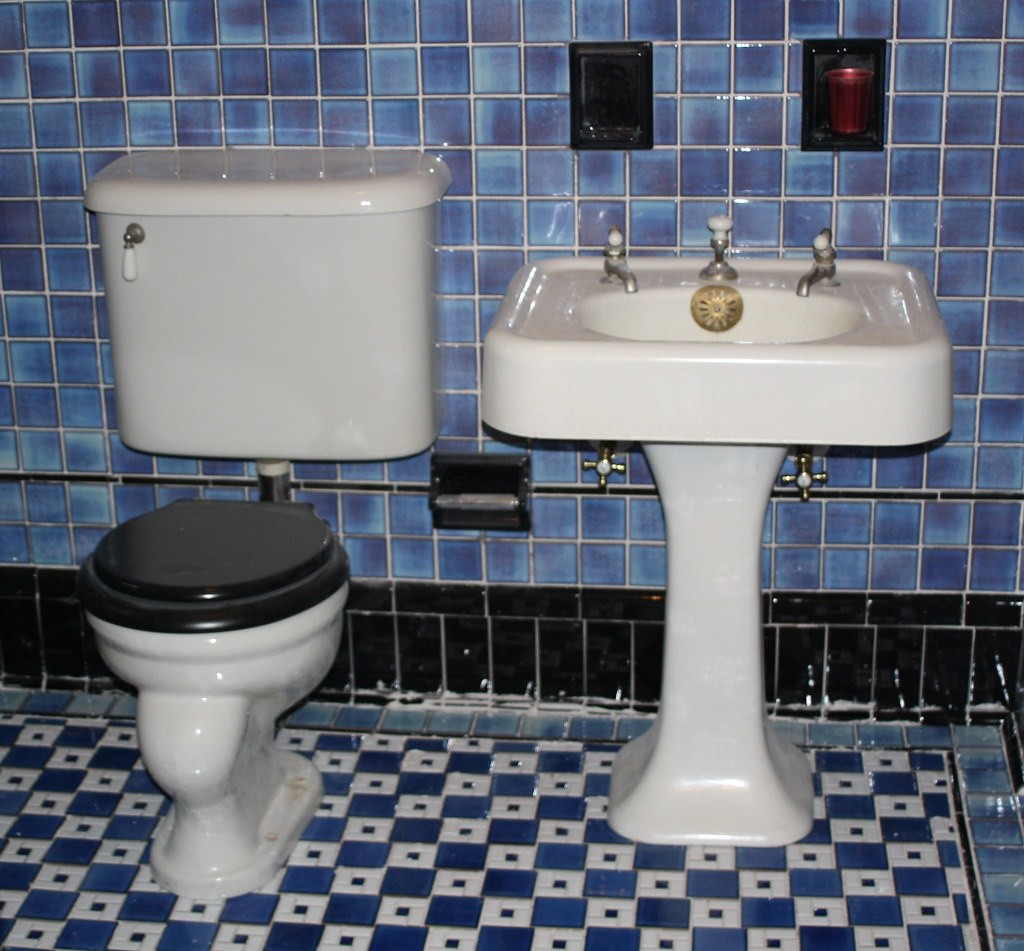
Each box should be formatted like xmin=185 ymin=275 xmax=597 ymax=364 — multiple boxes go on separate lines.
xmin=578 ymin=57 xmax=647 ymax=144
xmin=827 ymin=67 xmax=874 ymax=133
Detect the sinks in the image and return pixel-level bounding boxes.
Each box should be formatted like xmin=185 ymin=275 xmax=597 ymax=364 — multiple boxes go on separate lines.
xmin=479 ymin=255 xmax=954 ymax=449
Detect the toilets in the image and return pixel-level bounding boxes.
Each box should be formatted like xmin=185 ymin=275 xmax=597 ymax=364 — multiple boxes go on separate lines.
xmin=78 ymin=150 xmax=452 ymax=902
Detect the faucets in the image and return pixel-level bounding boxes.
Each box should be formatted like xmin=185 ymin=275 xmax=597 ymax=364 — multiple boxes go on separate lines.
xmin=795 ymin=228 xmax=841 ymax=297
xmin=603 ymin=223 xmax=639 ymax=293
